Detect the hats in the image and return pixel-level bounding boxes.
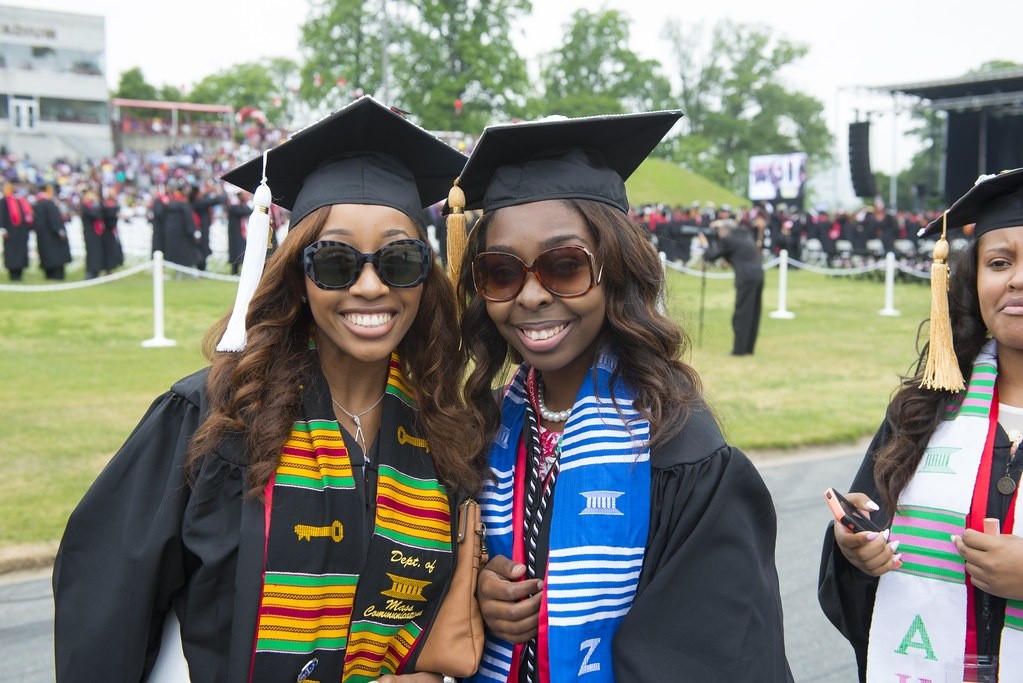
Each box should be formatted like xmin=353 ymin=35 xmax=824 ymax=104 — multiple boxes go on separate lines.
xmin=918 ymin=168 xmax=1023 ymax=393
xmin=440 ymin=110 xmax=684 ymax=332
xmin=215 ymin=95 xmax=474 ymax=352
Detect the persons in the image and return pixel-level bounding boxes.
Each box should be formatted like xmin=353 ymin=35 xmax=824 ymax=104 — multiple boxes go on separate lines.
xmin=50 ymin=94 xmax=472 ymax=683
xmin=818 ymin=167 xmax=1023 ymax=683
xmin=0 ymin=117 xmax=288 ymax=277
xmin=696 ymin=214 xmax=767 ymax=355
xmin=429 ymin=198 xmax=947 ymax=284
xmin=441 ymin=109 xmax=799 ymax=683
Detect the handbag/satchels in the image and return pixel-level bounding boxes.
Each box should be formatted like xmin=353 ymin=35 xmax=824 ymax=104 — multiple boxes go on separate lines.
xmin=413 ymin=498 xmax=485 ymax=677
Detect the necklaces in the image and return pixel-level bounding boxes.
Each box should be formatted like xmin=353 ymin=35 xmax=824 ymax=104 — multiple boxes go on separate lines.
xmin=330 ymin=392 xmax=384 ymax=463
xmin=536 ymin=374 xmax=571 ymax=423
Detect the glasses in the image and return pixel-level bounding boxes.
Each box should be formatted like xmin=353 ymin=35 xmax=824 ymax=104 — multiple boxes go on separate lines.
xmin=472 ymin=246 xmax=603 ymax=302
xmin=303 ymin=239 xmax=432 ymax=290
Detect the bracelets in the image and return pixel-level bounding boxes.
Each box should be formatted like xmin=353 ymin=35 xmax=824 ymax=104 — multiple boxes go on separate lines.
xmin=442 ymin=674 xmax=457 ymax=683
